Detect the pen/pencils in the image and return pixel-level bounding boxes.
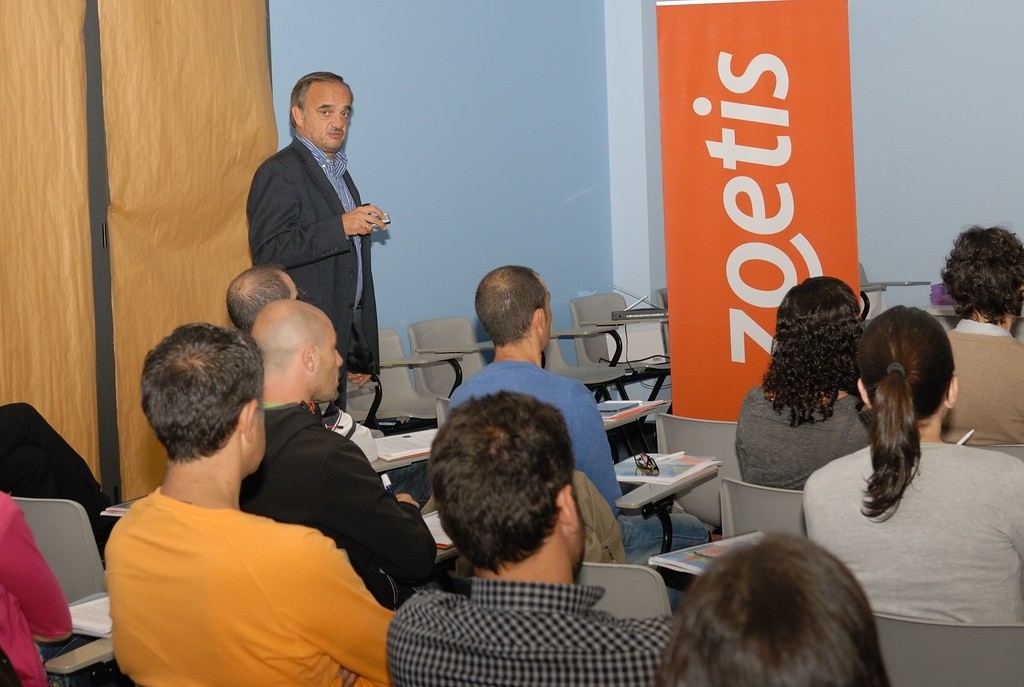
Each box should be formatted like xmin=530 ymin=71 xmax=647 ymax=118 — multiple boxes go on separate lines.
xmin=656 ymin=450 xmax=686 ymax=462
xmin=956 ymin=428 xmax=975 ymax=445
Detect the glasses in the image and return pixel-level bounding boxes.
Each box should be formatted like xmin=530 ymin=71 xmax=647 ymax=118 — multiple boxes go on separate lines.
xmin=635 ymin=453 xmax=659 ymax=472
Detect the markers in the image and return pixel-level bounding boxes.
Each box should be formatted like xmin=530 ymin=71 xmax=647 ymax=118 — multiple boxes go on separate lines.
xmin=381 ymin=473 xmax=397 ymax=500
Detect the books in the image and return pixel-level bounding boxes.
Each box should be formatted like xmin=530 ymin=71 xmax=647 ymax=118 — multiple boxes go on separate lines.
xmin=649 ymin=531 xmax=764 ymax=577
xmin=597 ymin=399 xmax=666 ymax=422
xmin=613 ymin=451 xmax=722 ymax=486
xmin=422 ymin=509 xmax=455 ymax=551
xmin=100 ymin=495 xmax=149 ymax=516
xmin=70 ymin=597 xmax=113 ymax=639
xmin=373 ymin=429 xmax=439 ymax=462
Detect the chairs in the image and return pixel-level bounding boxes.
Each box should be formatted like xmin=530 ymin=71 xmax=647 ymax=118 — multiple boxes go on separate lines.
xmin=9 ymin=262 xmax=1024 ymax=687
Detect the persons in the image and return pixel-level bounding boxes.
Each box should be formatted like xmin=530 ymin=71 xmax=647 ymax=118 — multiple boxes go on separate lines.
xmin=387 ymin=390 xmax=675 ymax=687
xmin=227 ymin=264 xmax=432 ymax=510
xmin=735 ymin=222 xmax=1024 ymax=627
xmin=450 ymin=265 xmax=711 ymax=616
xmin=105 ymin=322 xmax=396 ymax=687
xmin=0 ymin=491 xmax=96 ymax=687
xmin=246 ymin=72 xmax=388 ymax=413
xmin=0 ymin=402 xmax=121 ymax=570
xmin=239 ymin=300 xmax=472 ymax=611
xmin=657 ymin=534 xmax=890 ymax=687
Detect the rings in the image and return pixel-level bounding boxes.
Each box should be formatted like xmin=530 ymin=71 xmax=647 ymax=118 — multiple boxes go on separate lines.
xmin=363 ymin=223 xmax=369 ymax=229
